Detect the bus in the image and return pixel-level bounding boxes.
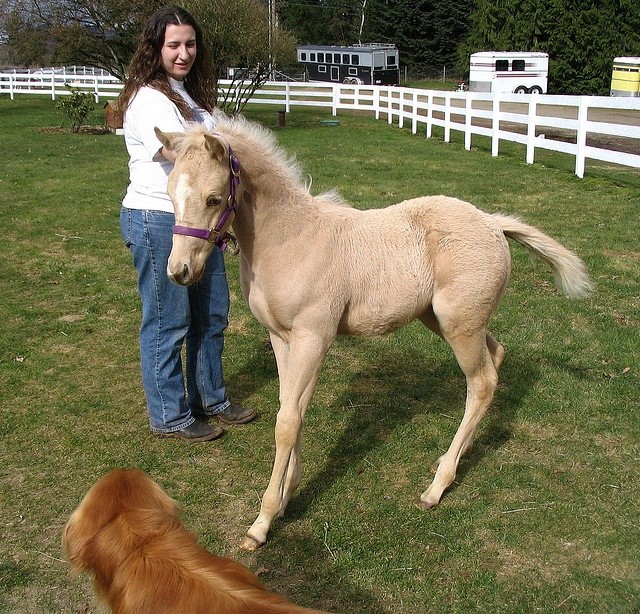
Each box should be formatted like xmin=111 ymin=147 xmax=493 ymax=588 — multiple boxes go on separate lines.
xmin=296 ymin=41 xmax=400 ymax=85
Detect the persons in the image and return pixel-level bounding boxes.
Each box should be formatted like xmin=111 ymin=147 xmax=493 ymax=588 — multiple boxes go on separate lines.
xmin=120 ymin=6 xmax=259 ymax=441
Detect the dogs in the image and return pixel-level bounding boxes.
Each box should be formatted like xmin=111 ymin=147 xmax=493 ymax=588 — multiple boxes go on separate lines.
xmin=62 ymin=467 xmax=336 ymax=612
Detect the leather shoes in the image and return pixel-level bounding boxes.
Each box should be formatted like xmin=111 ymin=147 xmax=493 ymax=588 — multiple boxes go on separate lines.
xmin=152 ymin=420 xmax=223 ymax=443
xmin=193 ymin=402 xmax=258 ymax=425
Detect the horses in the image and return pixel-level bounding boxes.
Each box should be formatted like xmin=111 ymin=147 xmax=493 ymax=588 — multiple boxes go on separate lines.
xmin=153 ymin=105 xmax=594 ymax=550
